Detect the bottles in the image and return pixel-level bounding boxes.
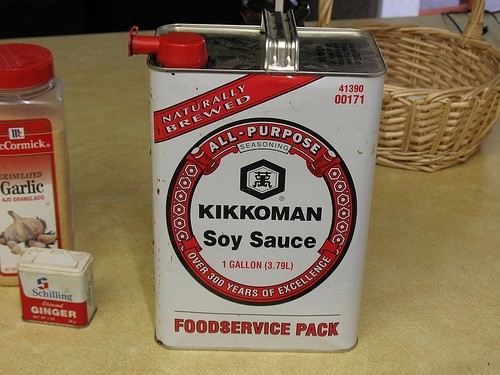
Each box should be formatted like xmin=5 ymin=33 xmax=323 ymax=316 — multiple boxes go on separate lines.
xmin=0 ymin=42 xmax=76 ymax=286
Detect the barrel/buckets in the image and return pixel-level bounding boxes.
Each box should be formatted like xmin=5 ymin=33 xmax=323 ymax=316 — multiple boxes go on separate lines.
xmin=128 ymin=9 xmax=387 ymax=354
